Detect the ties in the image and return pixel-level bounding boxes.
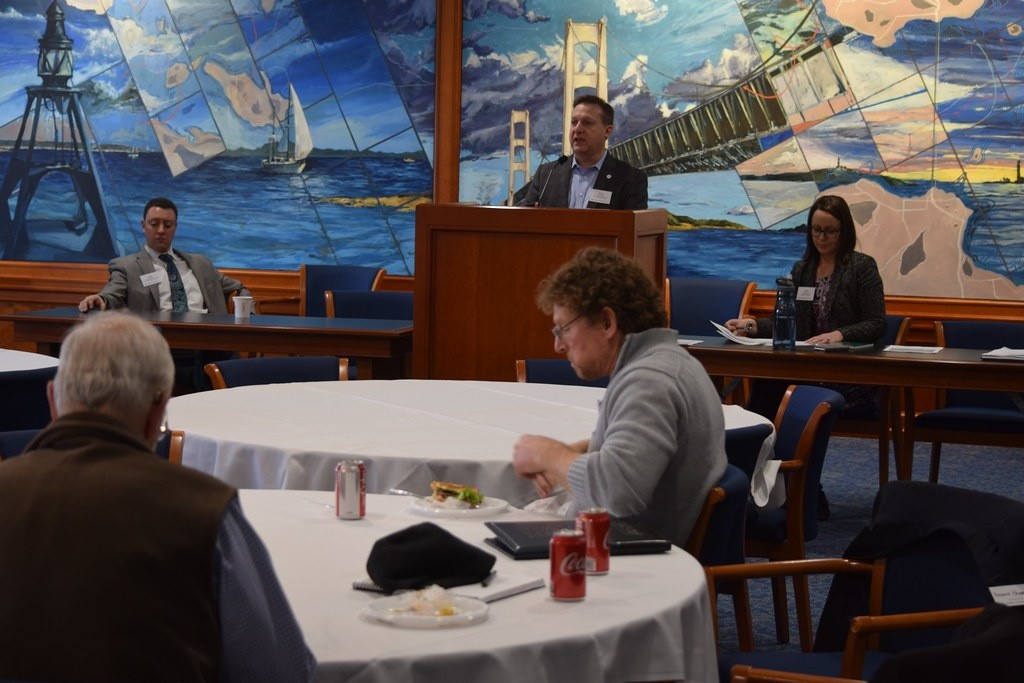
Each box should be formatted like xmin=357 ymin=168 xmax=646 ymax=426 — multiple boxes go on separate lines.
xmin=158 ymin=254 xmax=188 ymax=314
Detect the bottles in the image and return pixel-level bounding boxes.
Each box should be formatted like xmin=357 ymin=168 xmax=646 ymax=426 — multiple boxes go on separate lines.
xmin=772 ymin=278 xmax=796 ymax=349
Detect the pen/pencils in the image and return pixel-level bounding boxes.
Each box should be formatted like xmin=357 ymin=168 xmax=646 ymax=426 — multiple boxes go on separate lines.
xmin=735 ymin=327 xmax=752 ymax=331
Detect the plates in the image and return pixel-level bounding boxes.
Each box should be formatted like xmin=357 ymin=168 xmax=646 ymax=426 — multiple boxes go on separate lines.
xmin=364 ymin=596 xmax=489 ymax=628
xmin=411 ymin=496 xmax=507 ymax=517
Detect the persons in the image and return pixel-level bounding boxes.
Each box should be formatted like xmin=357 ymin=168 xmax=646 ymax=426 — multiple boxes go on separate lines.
xmin=725 ymin=195 xmax=886 ymax=519
xmin=513 ymin=94 xmax=648 ymax=209
xmin=0 ymin=313 xmax=318 ymax=682
xmin=79 ymin=197 xmax=256 ymax=397
xmin=513 ymin=246 xmax=728 ymax=546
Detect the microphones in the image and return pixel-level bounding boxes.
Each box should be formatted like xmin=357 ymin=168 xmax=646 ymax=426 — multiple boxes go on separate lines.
xmin=538 ymin=155 xmax=568 ymax=208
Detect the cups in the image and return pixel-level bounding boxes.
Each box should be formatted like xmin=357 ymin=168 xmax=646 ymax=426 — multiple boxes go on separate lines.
xmin=233 ymin=296 xmax=252 ymax=319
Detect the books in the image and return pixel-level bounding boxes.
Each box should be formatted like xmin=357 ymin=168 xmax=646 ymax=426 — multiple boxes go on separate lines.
xmin=352 ymin=572 xmax=545 ymax=602
xmin=982 ymin=347 xmax=1024 ymax=361
xmin=485 ymin=516 xmax=671 ymax=559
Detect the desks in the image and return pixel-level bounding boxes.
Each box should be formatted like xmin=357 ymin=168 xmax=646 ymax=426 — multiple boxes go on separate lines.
xmin=0 ymin=348 xmax=60 ymax=431
xmin=678 ymin=336 xmax=1024 ymax=483
xmin=237 ymin=488 xmax=719 ymax=683
xmin=1 ymin=307 xmax=413 ymax=380
xmin=161 ymin=380 xmax=787 ymax=512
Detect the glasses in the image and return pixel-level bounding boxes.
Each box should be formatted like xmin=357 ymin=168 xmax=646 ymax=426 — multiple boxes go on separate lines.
xmin=809 ymin=227 xmax=841 ymax=239
xmin=552 ymin=314 xmax=585 ymax=341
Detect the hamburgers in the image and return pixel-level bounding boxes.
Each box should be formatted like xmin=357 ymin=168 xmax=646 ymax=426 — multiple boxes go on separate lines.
xmin=431 ymin=480 xmax=482 ymax=509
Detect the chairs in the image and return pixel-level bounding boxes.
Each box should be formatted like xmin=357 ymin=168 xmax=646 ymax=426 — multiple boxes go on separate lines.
xmin=0 ymin=266 xmax=1023 ymax=683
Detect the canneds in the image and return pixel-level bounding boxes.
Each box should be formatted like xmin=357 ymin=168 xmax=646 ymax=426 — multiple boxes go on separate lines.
xmin=548 ymin=528 xmax=587 ymax=602
xmin=335 ymin=460 xmax=367 ymax=519
xmin=575 ymin=508 xmax=611 ymax=575
